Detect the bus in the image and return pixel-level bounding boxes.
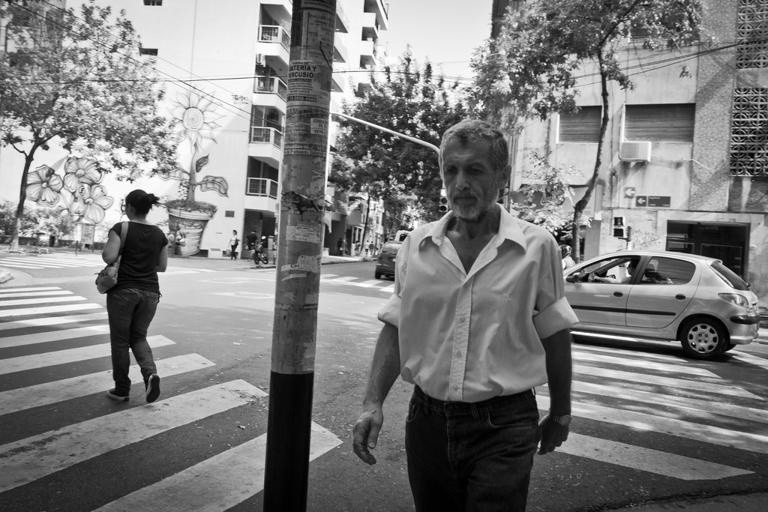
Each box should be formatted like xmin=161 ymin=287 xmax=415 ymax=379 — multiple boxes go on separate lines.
xmin=392 ymin=229 xmax=412 ymax=244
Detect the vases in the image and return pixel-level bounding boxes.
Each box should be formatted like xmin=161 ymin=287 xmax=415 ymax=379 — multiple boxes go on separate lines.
xmin=165 ymin=201 xmax=217 ymax=256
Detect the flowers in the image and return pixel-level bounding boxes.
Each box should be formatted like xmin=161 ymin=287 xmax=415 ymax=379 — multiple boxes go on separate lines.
xmin=25 ymin=154 xmax=113 ymax=241
xmin=156 ymin=90 xmax=228 ymax=210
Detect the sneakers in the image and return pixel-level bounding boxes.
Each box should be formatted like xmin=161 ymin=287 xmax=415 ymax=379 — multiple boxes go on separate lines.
xmin=145 ymin=374 xmax=161 ymax=403
xmin=107 ymin=389 xmax=129 ymax=402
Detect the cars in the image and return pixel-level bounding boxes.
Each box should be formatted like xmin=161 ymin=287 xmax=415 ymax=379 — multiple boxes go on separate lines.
xmin=561 ymin=247 xmax=764 ymax=360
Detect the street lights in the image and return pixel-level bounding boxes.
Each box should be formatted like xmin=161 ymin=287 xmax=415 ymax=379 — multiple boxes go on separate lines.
xmin=357 ymin=193 xmax=377 ymax=256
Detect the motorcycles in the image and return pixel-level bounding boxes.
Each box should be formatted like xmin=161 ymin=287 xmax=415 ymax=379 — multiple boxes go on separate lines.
xmin=250 ymin=236 xmax=269 ymax=265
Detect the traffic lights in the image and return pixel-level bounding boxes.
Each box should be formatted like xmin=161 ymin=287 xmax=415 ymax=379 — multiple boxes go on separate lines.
xmin=438 ymin=188 xmax=450 ymax=212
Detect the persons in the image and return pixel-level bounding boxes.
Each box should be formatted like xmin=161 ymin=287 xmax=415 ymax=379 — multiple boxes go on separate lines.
xmin=102 ymin=189 xmax=168 ymax=403
xmin=353 ymin=118 xmax=579 ymax=512
xmin=336 ymin=238 xmax=383 ymax=256
xmin=229 ymin=229 xmax=268 ymax=260
xmin=594 ymin=259 xmax=639 ymax=283
xmin=560 ymin=244 xmax=575 ymax=275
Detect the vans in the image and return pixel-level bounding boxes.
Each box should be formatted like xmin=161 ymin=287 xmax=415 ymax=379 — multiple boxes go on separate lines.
xmin=374 ymin=239 xmax=404 ymax=280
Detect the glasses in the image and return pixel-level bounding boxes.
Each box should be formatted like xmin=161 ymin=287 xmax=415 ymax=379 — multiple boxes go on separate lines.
xmin=121 ymin=205 xmax=132 ymax=212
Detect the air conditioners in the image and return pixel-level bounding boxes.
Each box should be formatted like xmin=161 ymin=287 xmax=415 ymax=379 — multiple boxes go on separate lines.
xmin=620 ymin=140 xmax=652 ymax=163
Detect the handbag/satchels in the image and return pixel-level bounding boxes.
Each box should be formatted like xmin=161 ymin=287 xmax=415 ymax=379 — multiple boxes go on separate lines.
xmin=95 ymin=262 xmax=119 ymax=294
